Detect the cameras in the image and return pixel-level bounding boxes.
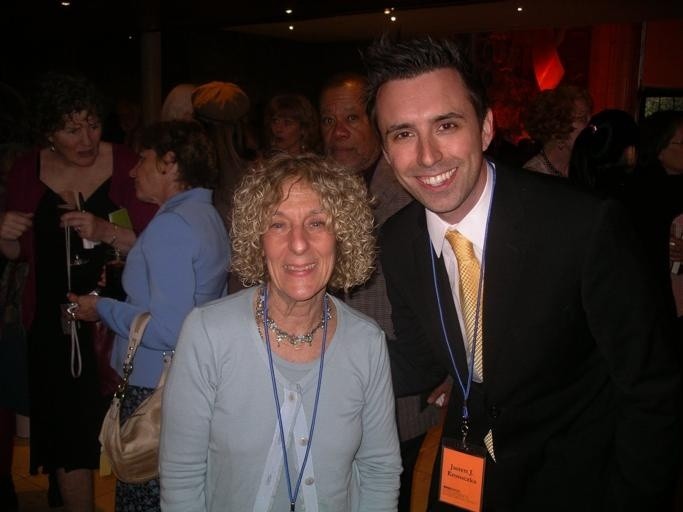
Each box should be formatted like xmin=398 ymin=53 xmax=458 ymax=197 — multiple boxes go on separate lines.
xmin=66 ymin=290 xmax=99 ymax=315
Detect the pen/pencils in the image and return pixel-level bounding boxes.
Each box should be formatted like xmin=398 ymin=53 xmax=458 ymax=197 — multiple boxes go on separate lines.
xmin=79 ymin=192 xmax=86 ymax=214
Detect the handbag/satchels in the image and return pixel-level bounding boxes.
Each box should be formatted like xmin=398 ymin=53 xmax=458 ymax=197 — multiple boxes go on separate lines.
xmin=98 ymin=309 xmax=175 ymax=485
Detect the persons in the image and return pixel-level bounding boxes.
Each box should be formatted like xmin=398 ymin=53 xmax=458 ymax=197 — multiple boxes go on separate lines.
xmin=267 ymin=74 xmax=411 ymax=338
xmin=159 ymin=151 xmax=403 ymax=511
xmin=521 ymin=82 xmax=682 ymax=311
xmin=375 ymin=35 xmax=659 ymax=511
xmin=0 ymin=79 xmax=259 ymax=512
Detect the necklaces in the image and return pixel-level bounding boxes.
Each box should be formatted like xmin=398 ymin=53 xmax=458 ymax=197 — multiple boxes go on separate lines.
xmin=256 ymin=281 xmax=334 ymax=345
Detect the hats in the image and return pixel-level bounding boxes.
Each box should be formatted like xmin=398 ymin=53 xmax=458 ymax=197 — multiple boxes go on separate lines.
xmin=190 ymin=80 xmax=252 ymax=120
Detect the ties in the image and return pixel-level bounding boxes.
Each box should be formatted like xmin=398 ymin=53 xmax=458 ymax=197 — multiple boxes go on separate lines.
xmin=444 ymin=228 xmax=497 ymax=464
